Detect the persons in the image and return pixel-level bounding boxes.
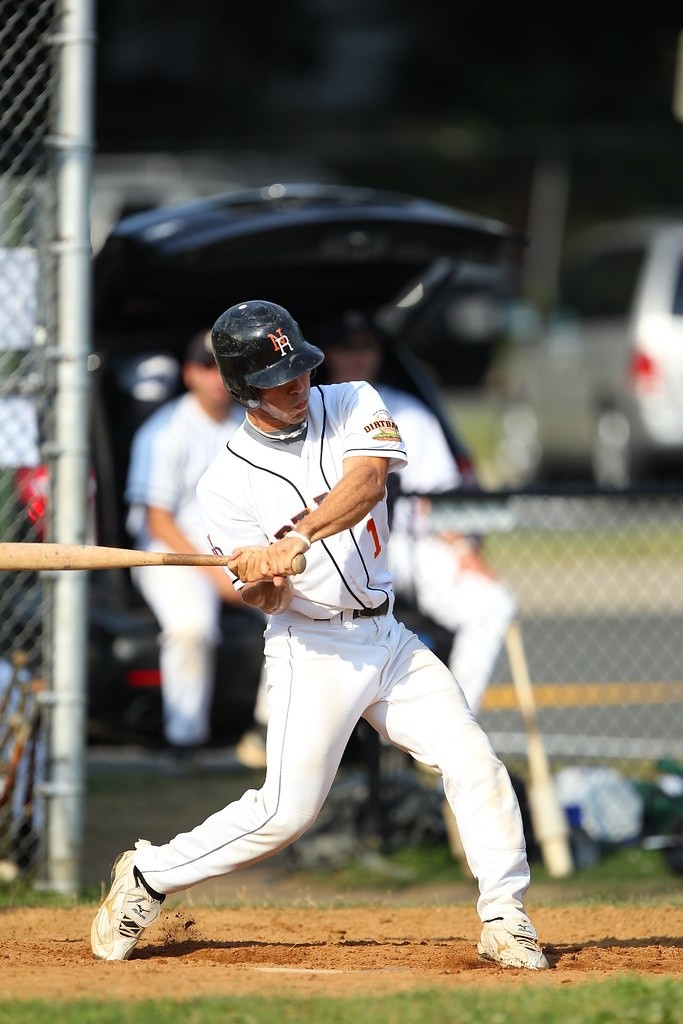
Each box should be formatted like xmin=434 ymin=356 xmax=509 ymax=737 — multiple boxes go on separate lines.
xmin=294 ymin=298 xmax=516 ymax=778
xmin=121 ymin=328 xmax=270 ymax=776
xmin=86 ymin=298 xmax=553 ymax=975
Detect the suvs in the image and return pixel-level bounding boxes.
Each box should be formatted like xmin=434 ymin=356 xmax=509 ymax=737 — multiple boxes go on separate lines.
xmin=3 ymin=181 xmax=532 ymax=744
xmin=489 ymin=220 xmax=682 ymax=496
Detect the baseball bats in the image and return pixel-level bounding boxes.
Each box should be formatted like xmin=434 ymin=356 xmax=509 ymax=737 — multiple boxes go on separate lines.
xmin=503 ymin=620 xmax=576 ymax=879
xmin=0 ymin=542 xmax=306 ymax=570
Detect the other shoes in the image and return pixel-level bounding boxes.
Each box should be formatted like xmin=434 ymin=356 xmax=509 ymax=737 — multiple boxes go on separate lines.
xmin=166 ymin=744 xmax=208 ymax=770
xmin=2 ymin=858 xmax=19 ymax=883
xmin=237 ymin=726 xmax=267 ymax=769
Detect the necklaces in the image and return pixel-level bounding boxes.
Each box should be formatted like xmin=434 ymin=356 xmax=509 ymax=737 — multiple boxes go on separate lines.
xmin=242 ymin=405 xmax=309 ymax=441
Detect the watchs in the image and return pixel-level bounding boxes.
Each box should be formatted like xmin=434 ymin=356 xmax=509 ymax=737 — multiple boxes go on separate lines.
xmin=281 ymin=529 xmax=313 ymax=550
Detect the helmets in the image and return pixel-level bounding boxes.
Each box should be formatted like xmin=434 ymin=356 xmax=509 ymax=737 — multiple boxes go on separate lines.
xmin=211 ymin=299 xmax=325 ymax=410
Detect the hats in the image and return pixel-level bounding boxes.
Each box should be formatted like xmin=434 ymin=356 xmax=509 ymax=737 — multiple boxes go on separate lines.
xmin=188 ymin=330 xmax=217 ymax=366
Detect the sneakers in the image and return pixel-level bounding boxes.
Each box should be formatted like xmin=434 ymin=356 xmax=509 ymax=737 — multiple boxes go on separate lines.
xmin=476 ymin=916 xmax=548 ymax=971
xmin=89 ymin=848 xmax=164 ymax=961
